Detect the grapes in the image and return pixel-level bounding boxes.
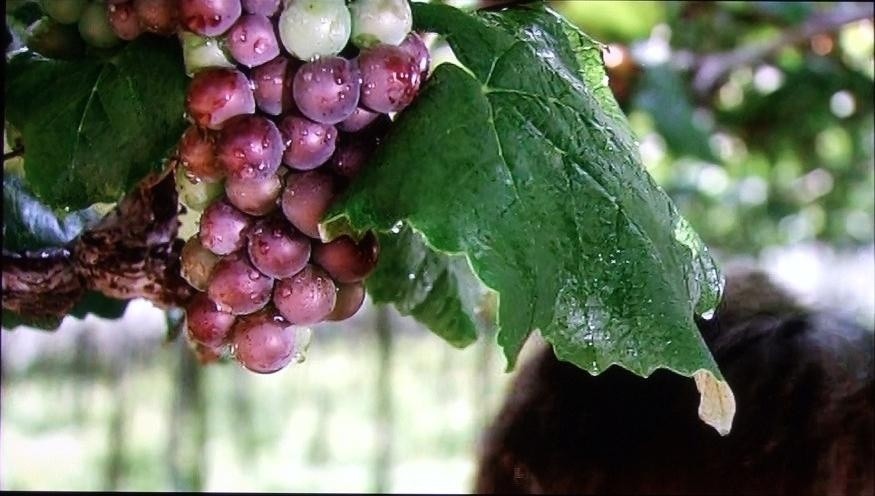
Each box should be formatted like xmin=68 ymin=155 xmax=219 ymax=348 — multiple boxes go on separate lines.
xmin=22 ymin=0 xmax=430 ymax=374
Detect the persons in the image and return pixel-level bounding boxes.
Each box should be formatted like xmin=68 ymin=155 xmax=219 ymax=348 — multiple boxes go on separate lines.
xmin=464 ymin=266 xmax=874 ymax=495
